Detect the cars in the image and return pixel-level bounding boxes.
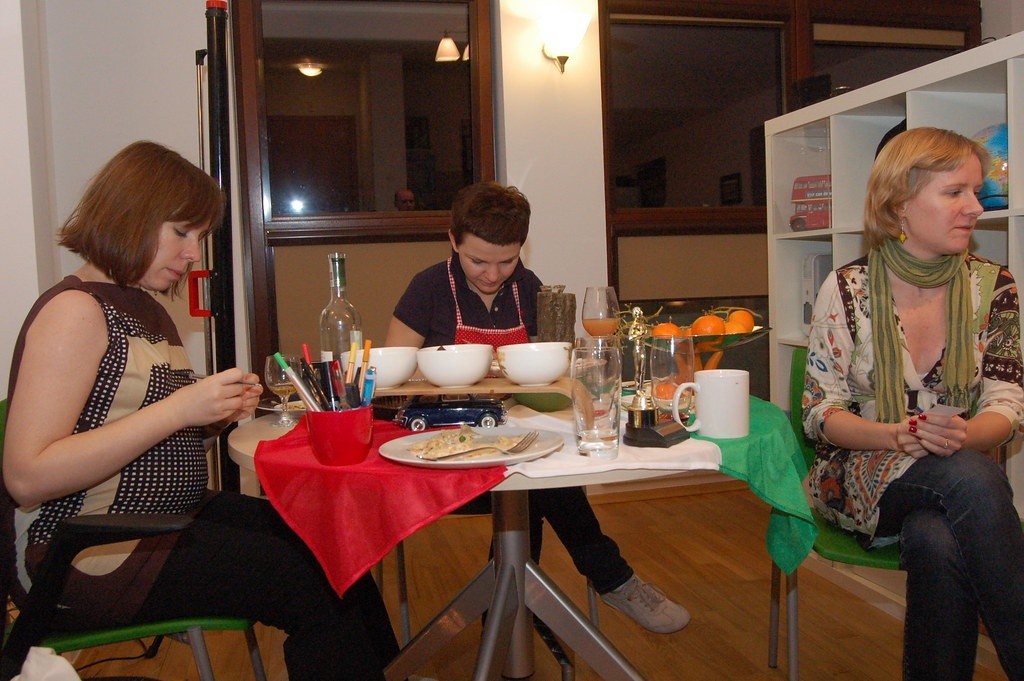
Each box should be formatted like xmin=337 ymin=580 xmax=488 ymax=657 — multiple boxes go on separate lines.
xmin=393 ymin=395 xmax=509 ymax=433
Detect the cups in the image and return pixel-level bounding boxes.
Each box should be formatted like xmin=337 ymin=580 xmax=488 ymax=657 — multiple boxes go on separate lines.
xmin=305 ymin=403 xmax=373 ymax=466
xmin=650 ymin=334 xmax=695 ymax=413
xmin=581 ymin=286 xmax=621 ymax=337
xmin=575 ymin=335 xmax=619 ymax=349
xmin=570 ymin=347 xmax=623 ymax=460
xmin=672 ymin=369 xmax=749 ymax=439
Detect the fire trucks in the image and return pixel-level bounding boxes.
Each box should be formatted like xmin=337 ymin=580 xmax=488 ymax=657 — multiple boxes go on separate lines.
xmin=789 ymin=174 xmax=832 ymax=233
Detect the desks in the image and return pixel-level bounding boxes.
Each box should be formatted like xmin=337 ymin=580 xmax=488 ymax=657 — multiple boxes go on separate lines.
xmin=228 ymin=377 xmax=814 ymax=681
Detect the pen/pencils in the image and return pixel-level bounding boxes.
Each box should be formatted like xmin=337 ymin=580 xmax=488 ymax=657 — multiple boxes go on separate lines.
xmin=273 ymin=340 xmax=375 ymax=412
xmin=172 ymin=372 xmax=261 ymax=387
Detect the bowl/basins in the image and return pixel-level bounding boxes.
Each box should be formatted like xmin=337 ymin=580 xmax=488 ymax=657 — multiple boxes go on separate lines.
xmin=497 ymin=341 xmax=572 ymax=386
xmin=417 ymin=344 xmax=493 ymax=388
xmin=339 ymin=346 xmax=419 ymax=391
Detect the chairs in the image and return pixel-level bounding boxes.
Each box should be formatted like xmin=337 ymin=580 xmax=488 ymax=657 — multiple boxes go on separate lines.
xmin=767 ymin=348 xmax=921 ymax=681
xmin=0 ymin=396 xmax=267 ymax=681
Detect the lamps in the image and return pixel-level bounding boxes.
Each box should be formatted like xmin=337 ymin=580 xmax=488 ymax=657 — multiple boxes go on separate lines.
xmin=433 ymin=28 xmax=460 ymax=62
xmin=540 ymin=41 xmax=574 ymax=73
xmin=293 ymin=54 xmax=327 ymax=78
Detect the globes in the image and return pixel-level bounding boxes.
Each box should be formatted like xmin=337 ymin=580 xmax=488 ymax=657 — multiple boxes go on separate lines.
xmin=971 ymin=122 xmax=1008 ymax=211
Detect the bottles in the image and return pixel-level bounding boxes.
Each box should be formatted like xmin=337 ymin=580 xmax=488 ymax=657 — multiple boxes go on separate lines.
xmin=319 ymin=252 xmax=361 ymax=360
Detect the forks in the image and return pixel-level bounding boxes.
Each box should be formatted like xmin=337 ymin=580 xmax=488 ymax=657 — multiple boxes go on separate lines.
xmin=416 ymin=430 xmax=539 ymax=460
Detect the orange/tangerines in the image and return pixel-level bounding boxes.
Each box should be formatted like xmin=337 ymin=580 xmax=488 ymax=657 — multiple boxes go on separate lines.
xmin=652 ymin=310 xmax=754 ymax=337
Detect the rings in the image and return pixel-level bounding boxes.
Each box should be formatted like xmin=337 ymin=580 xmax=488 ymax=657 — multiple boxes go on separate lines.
xmin=944 ymin=438 xmax=949 ymax=449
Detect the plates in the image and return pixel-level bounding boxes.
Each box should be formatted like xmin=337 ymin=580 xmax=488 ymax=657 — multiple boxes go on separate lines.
xmin=378 ymin=427 xmax=564 ymax=467
xmin=257 ymin=396 xmax=306 ymax=417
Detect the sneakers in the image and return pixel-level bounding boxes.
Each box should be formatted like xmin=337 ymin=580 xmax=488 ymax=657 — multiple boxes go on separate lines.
xmin=600 ymin=571 xmax=691 ymax=634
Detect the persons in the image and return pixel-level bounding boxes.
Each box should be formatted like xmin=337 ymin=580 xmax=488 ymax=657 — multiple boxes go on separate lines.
xmin=381 ymin=181 xmax=692 ymax=635
xmin=0 ymin=141 xmax=409 ymax=681
xmin=801 ymin=127 xmax=1024 ymax=681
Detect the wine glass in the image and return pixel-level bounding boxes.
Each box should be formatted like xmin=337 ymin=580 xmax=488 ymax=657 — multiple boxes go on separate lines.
xmin=265 ymin=355 xmax=302 ymax=426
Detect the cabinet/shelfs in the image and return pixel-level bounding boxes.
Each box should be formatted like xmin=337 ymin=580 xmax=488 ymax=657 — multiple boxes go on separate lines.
xmin=764 ymin=31 xmax=1023 ymax=516
